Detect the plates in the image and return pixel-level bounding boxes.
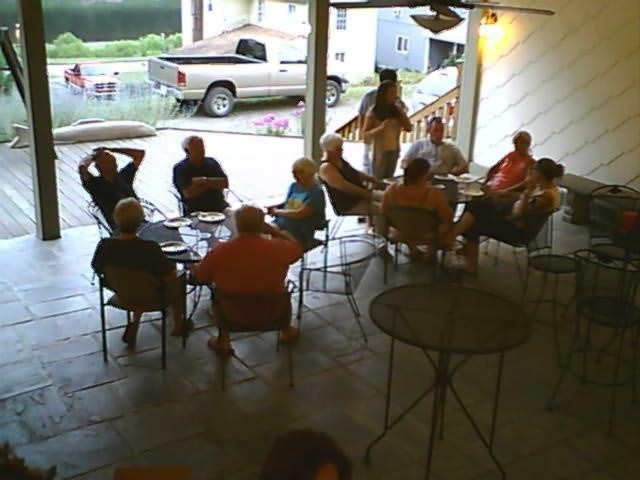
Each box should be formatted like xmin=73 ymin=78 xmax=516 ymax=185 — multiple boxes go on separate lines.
xmin=162 ymin=217 xmax=193 ymax=229
xmin=159 ymin=240 xmax=188 ymax=253
xmin=448 ymin=171 xmax=485 ymax=183
xmin=196 ymin=211 xmax=226 ymax=223
xmin=424 ymin=180 xmax=447 ymax=190
xmin=434 ymin=170 xmax=456 ymax=181
xmin=458 ymin=187 xmax=486 ymax=198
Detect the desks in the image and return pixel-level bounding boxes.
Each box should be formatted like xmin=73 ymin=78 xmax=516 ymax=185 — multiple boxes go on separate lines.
xmin=363 ymin=283 xmax=531 ymax=480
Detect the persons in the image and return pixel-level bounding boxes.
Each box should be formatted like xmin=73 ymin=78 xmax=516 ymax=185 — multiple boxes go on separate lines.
xmin=401 ymin=117 xmax=470 ymax=176
xmin=440 ymin=159 xmax=563 ymax=275
xmin=261 ymin=429 xmax=352 ymax=479
xmin=262 ymin=157 xmax=326 ymax=282
xmin=173 ymin=135 xmax=233 ymax=218
xmin=90 ymin=196 xmax=194 ymax=345
xmin=191 ymin=205 xmax=304 ymax=352
xmin=383 ymin=157 xmax=452 ymax=258
xmin=363 ymin=80 xmax=412 ymax=180
xmin=319 ymin=131 xmax=393 ymax=261
xmin=357 ymin=69 xmax=400 ymax=176
xmin=79 ymin=147 xmax=151 ymax=237
xmin=456 ymin=130 xmax=536 ymax=256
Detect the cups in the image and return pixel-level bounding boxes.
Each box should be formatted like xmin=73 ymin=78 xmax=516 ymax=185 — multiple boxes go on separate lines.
xmin=623 ymin=209 xmax=638 ymax=231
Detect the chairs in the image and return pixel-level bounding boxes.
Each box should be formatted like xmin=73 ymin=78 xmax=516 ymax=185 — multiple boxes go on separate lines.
xmin=313 ymin=142 xmax=569 ymax=268
xmin=505 ymin=184 xmax=640 ymax=440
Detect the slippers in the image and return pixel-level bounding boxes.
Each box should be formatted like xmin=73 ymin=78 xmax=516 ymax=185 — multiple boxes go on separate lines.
xmin=278 ymin=325 xmax=298 ymax=343
xmin=122 ymin=324 xmax=135 ymax=341
xmin=170 ymin=318 xmax=193 ymax=336
xmin=207 ymin=334 xmax=234 ymax=357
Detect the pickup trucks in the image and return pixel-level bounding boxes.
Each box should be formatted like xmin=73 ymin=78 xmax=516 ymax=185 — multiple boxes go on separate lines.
xmin=64 ymin=60 xmax=120 ymax=105
xmin=148 ymin=35 xmax=354 ymax=119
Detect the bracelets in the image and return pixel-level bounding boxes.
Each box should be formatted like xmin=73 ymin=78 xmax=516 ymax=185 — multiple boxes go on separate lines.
xmin=203 ymin=176 xmax=208 ymax=183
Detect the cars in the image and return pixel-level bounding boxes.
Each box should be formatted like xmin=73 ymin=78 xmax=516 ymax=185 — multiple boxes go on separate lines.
xmin=412 ymin=64 xmax=461 ymax=131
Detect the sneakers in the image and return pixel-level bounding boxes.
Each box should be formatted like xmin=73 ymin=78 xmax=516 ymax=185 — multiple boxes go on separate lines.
xmin=453 ymin=246 xmax=479 ymax=277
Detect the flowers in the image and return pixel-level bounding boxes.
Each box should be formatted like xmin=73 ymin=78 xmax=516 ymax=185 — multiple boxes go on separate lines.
xmin=254 ymin=100 xmax=306 ymax=137
